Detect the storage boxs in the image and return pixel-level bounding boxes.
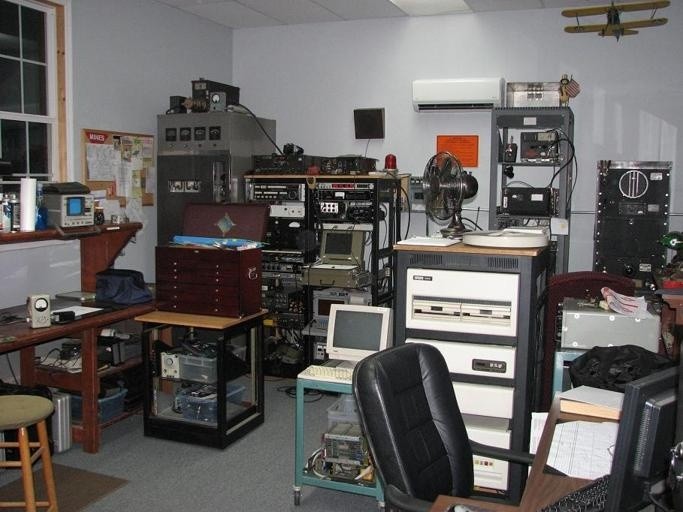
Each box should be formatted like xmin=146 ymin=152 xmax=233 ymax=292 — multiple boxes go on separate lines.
xmin=327 ymin=397 xmax=359 ymax=434
xmin=71 ymin=388 xmax=128 ymax=421
xmin=506 ymin=80 xmax=563 ymax=108
xmin=155 ymin=204 xmax=270 ymax=319
xmin=178 ymin=384 xmax=246 ymax=424
xmin=179 ymin=344 xmax=246 ymax=381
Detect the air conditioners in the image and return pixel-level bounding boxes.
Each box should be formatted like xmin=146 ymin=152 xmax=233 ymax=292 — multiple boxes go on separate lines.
xmin=412 ymin=78 xmax=505 ymax=113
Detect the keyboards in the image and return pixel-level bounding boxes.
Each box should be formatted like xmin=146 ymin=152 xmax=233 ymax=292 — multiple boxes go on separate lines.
xmin=536 ymin=473 xmax=611 ymax=512
xmin=298 ymin=365 xmax=354 ymax=385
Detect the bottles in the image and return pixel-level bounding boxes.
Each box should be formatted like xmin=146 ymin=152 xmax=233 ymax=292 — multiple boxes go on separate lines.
xmin=0 ymin=193 xmax=23 ymax=233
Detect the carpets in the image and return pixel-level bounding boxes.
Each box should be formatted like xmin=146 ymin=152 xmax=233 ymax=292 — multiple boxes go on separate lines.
xmin=0 ymin=461 xmax=130 ymax=512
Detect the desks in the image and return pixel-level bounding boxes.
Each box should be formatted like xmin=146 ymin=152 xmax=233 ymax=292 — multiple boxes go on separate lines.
xmin=0 ymin=222 xmax=163 ymax=454
xmin=136 ymin=307 xmax=269 ymax=449
xmin=552 ymin=348 xmax=587 ymax=406
xmin=651 ymin=268 xmax=683 ymax=361
xmin=431 ymin=392 xmax=620 ymax=511
xmin=293 ymin=358 xmax=385 ymax=511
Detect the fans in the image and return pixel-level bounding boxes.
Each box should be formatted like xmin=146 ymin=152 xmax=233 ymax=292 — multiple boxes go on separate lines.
xmin=423 ymin=151 xmax=475 ymax=241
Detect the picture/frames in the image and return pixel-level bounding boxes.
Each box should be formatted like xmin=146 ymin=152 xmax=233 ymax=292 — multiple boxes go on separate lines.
xmin=82 ymin=128 xmax=154 ymax=207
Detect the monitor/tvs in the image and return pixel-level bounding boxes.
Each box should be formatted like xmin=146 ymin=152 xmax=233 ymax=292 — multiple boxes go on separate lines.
xmin=312 ymin=288 xmax=348 ymax=330
xmin=325 ymin=304 xmax=394 ymax=370
xmin=608 ymin=365 xmax=683 ymax=511
xmin=320 ymin=230 xmax=364 ymax=264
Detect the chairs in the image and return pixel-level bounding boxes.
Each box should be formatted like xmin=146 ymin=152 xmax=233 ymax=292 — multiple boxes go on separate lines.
xmin=355 ymin=344 xmax=536 ymax=511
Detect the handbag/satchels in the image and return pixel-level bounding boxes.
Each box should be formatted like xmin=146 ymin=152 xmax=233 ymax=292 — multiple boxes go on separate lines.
xmin=94 ymin=268 xmax=154 ymax=305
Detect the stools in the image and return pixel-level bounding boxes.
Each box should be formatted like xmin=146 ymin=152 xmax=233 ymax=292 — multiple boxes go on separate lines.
xmin=0 ymin=395 xmax=58 ymax=512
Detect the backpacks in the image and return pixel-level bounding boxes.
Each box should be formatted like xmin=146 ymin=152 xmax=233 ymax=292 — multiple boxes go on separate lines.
xmin=568 ymin=344 xmax=679 ymax=395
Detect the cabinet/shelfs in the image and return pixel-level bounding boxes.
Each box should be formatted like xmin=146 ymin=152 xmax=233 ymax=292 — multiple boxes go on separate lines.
xmin=394 ymin=239 xmax=550 ymax=506
xmin=489 ymin=106 xmax=575 ymax=273
xmin=245 ymin=172 xmax=400 ymax=392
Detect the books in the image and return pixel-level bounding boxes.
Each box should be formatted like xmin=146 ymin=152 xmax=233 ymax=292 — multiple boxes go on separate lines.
xmin=555 ymin=383 xmax=625 ymax=420
